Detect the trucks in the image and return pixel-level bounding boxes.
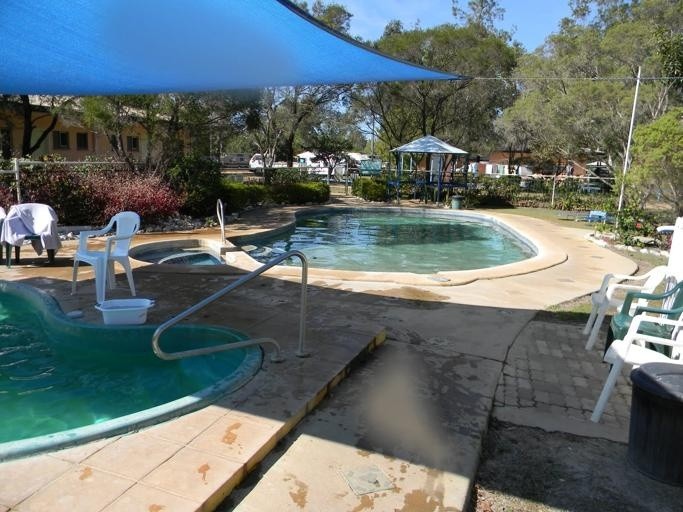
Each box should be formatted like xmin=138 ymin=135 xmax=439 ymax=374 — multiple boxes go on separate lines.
xmin=247 ymin=153 xmax=299 ymax=176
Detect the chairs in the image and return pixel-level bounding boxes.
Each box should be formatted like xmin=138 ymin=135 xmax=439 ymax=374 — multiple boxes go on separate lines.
xmin=0 ymin=201 xmax=61 ymax=270
xmin=582 ymin=264 xmax=683 ymax=425
xmin=70 ymin=210 xmax=142 ymax=305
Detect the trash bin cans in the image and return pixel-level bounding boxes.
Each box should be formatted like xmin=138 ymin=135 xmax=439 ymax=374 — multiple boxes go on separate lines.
xmin=624 ymin=362 xmax=683 ymax=487
xmin=452 ymin=196 xmax=465 ymax=209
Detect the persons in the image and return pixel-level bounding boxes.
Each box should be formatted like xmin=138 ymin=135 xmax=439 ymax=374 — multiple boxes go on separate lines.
xmin=564 ymin=161 xmax=574 ymax=176
xmin=515 ymin=157 xmax=529 ymax=188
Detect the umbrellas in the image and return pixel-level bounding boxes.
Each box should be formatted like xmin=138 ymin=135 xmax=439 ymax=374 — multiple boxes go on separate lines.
xmin=0 ymin=0 xmax=473 ymax=99
xmin=387 ymin=133 xmax=469 ymax=205
